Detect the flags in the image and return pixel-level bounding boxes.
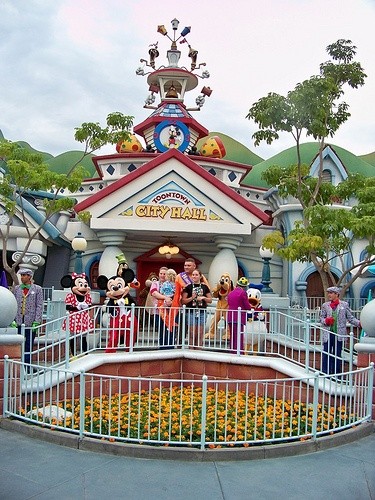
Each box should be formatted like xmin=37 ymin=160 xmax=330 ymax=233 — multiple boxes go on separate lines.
xmin=179 ymin=39 xmax=186 ymax=44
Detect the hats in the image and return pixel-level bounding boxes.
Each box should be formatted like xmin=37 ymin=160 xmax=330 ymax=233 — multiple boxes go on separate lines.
xmin=236 ymin=277 xmax=249 ymax=286
xmin=326 ymin=287 xmax=342 ymax=295
xmin=17 ymin=269 xmax=33 ymax=275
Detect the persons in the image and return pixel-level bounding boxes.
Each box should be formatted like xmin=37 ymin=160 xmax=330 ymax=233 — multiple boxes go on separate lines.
xmin=9 ymin=269 xmax=44 ymax=375
xmin=227 ymin=277 xmax=251 ymax=355
xmin=159 ymin=269 xmax=178 ymax=310
xmin=175 ymin=258 xmax=210 ymax=350
xmin=181 ymin=269 xmax=212 ymax=351
xmin=150 ymin=267 xmax=176 ymax=350
xmin=320 ymin=287 xmax=362 ymax=381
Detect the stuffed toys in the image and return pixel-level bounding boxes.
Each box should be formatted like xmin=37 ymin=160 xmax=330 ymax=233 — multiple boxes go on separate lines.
xmin=116 ymin=253 xmax=140 ymax=345
xmin=244 ymin=283 xmax=267 ymax=356
xmin=205 ymin=274 xmax=234 ymax=341
xmin=138 ymin=272 xmax=159 ymax=328
xmin=60 ymin=273 xmax=94 ymax=357
xmin=97 ymin=269 xmax=138 ymax=353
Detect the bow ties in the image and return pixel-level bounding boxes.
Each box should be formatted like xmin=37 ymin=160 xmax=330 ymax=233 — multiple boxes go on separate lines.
xmin=20 ymin=283 xmax=30 ymax=290
xmin=330 ymin=299 xmax=339 ymax=309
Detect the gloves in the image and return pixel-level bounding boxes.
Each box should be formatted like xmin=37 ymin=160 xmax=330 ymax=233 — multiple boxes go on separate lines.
xmin=324 ymin=317 xmax=335 ymax=326
xmin=10 ymin=322 xmax=17 ymax=328
xmin=31 ymin=322 xmax=39 ymax=333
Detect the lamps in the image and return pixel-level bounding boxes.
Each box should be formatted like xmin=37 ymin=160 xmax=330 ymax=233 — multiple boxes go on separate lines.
xmin=259 ymin=244 xmax=274 ymax=294
xmin=72 ymin=232 xmax=87 ymax=273
xmin=159 ymin=238 xmax=179 ymax=258
